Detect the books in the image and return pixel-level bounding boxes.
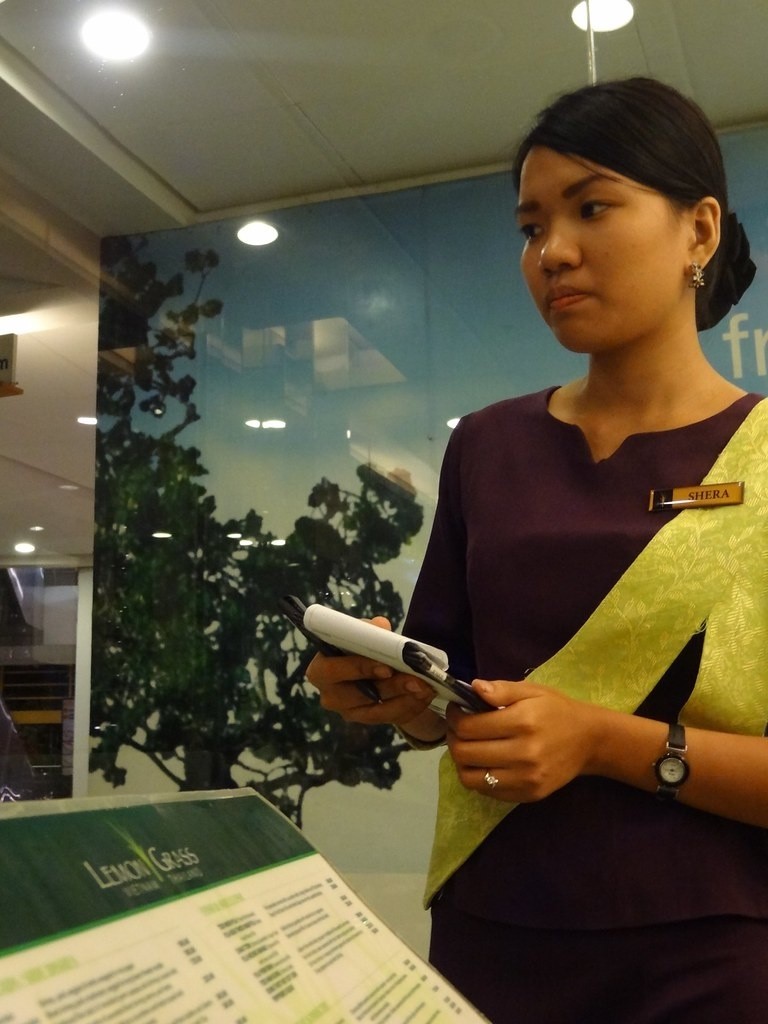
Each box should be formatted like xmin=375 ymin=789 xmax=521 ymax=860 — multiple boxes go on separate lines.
xmin=303 ymin=604 xmax=505 ymax=721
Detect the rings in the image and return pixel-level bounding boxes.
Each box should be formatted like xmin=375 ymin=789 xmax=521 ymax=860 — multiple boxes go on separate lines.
xmin=484 ymin=768 xmax=498 ymax=795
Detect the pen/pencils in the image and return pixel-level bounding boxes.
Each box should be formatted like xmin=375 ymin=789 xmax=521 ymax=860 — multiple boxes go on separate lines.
xmin=273 ymin=593 xmax=386 ymax=706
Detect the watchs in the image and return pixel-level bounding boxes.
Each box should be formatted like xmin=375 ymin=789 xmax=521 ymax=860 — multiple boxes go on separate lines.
xmin=651 ymin=723 xmax=691 ymax=802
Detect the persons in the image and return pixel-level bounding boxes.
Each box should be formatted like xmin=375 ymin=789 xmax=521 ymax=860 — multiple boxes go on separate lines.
xmin=305 ymin=77 xmax=768 ymax=1024
